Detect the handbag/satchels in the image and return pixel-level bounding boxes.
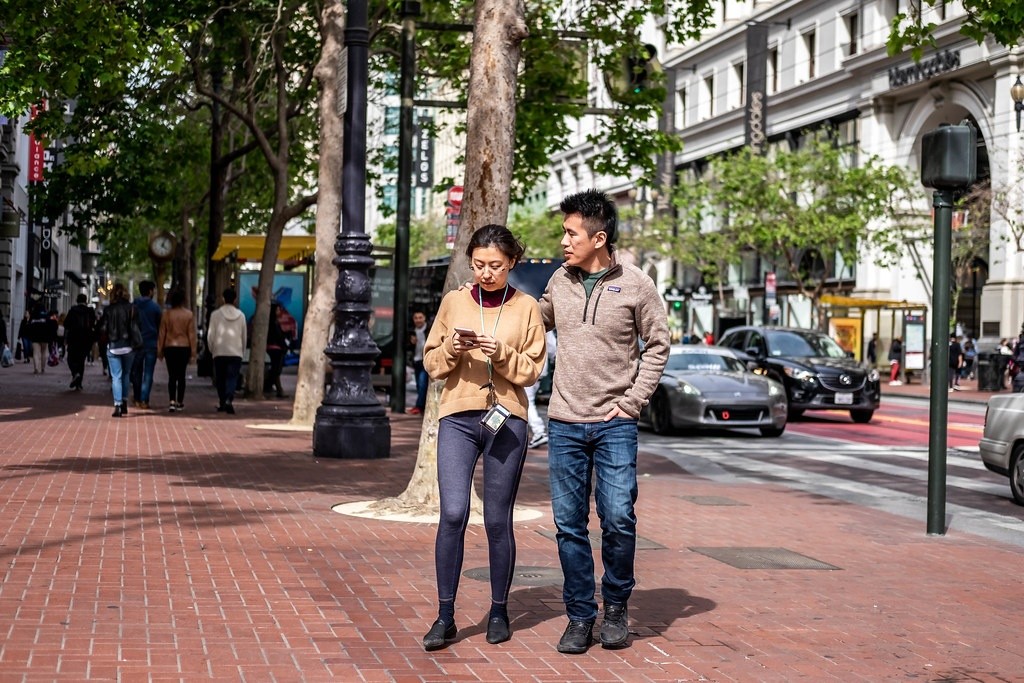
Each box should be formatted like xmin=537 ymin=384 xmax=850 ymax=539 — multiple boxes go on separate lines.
xmin=48 ymin=346 xmax=59 ymax=367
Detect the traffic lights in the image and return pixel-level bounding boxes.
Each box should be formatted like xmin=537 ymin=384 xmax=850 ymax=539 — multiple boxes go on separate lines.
xmin=672 ymin=300 xmax=682 ymax=312
xmin=625 ymin=42 xmax=656 ymax=96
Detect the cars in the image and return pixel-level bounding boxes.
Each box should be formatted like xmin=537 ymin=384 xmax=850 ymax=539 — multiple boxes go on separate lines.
xmin=978 ymin=392 xmax=1024 ymax=508
xmin=640 ymin=343 xmax=789 ymax=438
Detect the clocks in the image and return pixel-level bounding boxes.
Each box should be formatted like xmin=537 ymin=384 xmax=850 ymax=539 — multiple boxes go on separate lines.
xmin=146 ymin=230 xmax=177 ymax=262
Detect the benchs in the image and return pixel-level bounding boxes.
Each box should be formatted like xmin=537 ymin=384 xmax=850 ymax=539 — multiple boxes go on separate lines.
xmin=871 ymin=363 xmax=913 ymax=384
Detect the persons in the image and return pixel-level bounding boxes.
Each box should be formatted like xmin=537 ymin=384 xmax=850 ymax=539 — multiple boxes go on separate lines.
xmin=251 ymin=298 xmax=295 ymax=400
xmin=423 ymin=224 xmax=547 ymax=648
xmin=407 ymin=310 xmax=432 ymax=415
xmin=96 ymin=284 xmax=134 ymax=417
xmin=63 ymin=294 xmax=95 ymax=390
xmin=157 ymin=288 xmax=197 ymax=412
xmin=947 ymin=332 xmax=979 ymax=393
xmin=459 ymin=188 xmax=671 ymax=653
xmin=683 ymin=330 xmax=714 ymax=345
xmin=867 ymin=333 xmax=877 ymax=362
xmin=888 ymin=336 xmax=903 ymax=386
xmin=18 ymin=305 xmax=112 ymax=378
xmin=132 ymin=280 xmax=162 ymax=409
xmin=207 ymin=288 xmax=247 ymax=415
xmin=1000 ymin=322 xmax=1024 ymax=389
xmin=0 ymin=309 xmax=9 ymax=362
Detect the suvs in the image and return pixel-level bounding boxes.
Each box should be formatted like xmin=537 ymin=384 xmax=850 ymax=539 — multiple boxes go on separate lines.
xmin=715 ymin=324 xmax=881 ymax=424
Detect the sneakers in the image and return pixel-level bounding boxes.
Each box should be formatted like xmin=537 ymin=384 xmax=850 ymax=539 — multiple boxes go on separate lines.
xmin=557 ymin=621 xmax=594 ymax=653
xmin=527 ymin=434 xmax=551 ymax=449
xmin=601 ymin=601 xmax=629 ymax=643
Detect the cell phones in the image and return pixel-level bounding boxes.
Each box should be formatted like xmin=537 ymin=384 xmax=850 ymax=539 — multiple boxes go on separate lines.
xmin=454 ymin=328 xmax=479 ymax=345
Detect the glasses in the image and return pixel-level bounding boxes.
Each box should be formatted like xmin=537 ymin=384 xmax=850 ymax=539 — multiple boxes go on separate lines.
xmin=468 ymin=262 xmax=511 ymax=275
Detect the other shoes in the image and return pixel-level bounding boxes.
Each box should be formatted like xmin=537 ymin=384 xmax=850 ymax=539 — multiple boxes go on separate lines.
xmin=141 ymin=401 xmax=150 ymax=408
xmin=69 ymin=373 xmax=81 ymax=389
xmin=111 ymin=407 xmax=122 ymax=417
xmin=76 ymin=382 xmax=83 ymax=391
xmin=889 ymin=381 xmax=898 ymax=386
xmin=217 ymin=402 xmax=225 ymax=412
xmin=177 ymin=404 xmax=184 ymax=411
xmin=123 ymin=402 xmax=128 ymax=414
xmin=276 ymin=390 xmax=290 ymax=400
xmin=169 ymin=400 xmax=176 ymax=412
xmin=948 ymin=389 xmax=954 ymax=393
xmin=422 ymin=620 xmax=457 ymax=648
xmin=225 ymin=394 xmax=236 ymax=414
xmin=486 ymin=615 xmax=510 ymax=642
xmin=406 ymin=406 xmax=419 ymax=415
